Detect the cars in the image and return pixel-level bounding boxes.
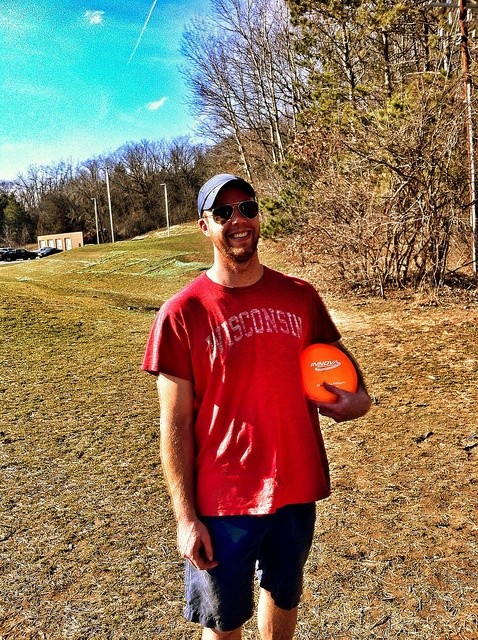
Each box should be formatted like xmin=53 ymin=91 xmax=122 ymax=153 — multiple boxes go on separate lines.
xmin=0 ymin=247 xmax=12 ymax=251
xmin=38 ymin=248 xmax=63 ymax=258
xmin=3 ymin=250 xmax=16 ymax=254
xmin=35 ymin=247 xmax=51 ymax=256
xmin=0 ymin=251 xmax=9 ymax=260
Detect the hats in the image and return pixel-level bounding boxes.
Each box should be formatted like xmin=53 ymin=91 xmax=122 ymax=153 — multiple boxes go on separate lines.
xmin=196 ymin=175 xmax=255 ymax=217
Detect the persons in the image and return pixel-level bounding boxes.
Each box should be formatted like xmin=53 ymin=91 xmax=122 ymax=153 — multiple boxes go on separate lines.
xmin=141 ymin=173 xmax=372 ymax=640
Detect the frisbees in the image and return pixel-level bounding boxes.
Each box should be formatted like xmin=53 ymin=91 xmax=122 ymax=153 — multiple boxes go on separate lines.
xmin=300 ymin=343 xmax=358 ymax=404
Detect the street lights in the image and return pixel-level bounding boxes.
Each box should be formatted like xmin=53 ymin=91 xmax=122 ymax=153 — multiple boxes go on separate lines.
xmin=98 ymin=167 xmax=115 ymax=244
xmin=90 ymin=197 xmax=100 ymax=244
xmin=159 ymin=183 xmax=170 ymax=238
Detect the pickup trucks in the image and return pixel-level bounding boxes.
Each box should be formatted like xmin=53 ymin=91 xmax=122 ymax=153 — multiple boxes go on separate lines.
xmin=3 ymin=249 xmax=37 ymax=262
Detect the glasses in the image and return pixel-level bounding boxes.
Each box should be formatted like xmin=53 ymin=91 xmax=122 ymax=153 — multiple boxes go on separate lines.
xmin=212 ymin=201 xmax=258 ymax=224
